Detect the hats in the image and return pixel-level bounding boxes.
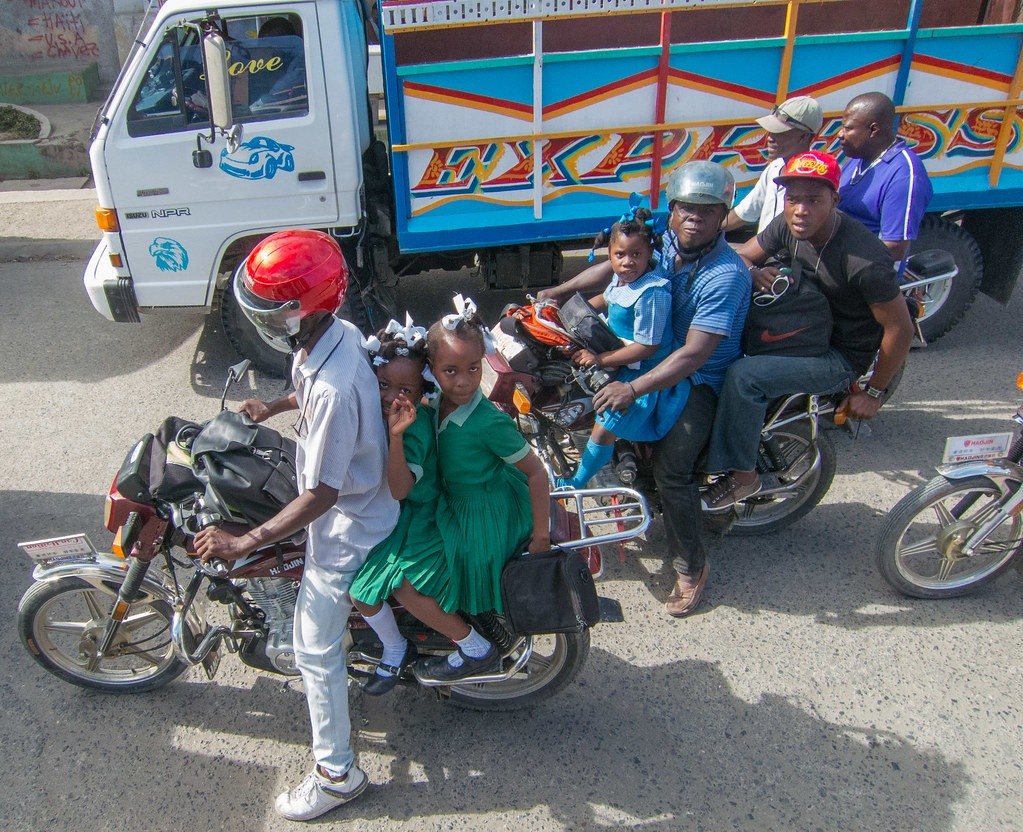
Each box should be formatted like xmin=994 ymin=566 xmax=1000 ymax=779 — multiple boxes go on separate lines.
xmin=773 ymin=149 xmax=841 ymax=190
xmin=754 ymin=95 xmax=822 ymax=134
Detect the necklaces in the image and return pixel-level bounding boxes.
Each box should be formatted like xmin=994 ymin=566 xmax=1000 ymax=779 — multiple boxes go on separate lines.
xmin=794 ymin=212 xmax=837 ymax=272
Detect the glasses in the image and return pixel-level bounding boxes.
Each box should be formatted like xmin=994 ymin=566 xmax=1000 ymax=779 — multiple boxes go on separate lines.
xmin=753 ymin=275 xmax=790 ymax=306
xmin=771 ymin=104 xmax=813 ymax=131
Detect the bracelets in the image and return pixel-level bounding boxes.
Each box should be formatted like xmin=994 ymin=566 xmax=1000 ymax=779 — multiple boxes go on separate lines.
xmin=628 ymin=383 xmax=636 ymax=399
xmin=748 ymin=266 xmax=758 ymax=271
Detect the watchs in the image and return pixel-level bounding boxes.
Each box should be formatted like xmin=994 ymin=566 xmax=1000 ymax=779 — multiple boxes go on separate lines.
xmin=865 ymin=382 xmax=888 ymax=398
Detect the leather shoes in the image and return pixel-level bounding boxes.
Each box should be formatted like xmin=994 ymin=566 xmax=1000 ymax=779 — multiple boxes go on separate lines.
xmin=363 ymin=637 xmax=417 ymax=696
xmin=430 ymin=642 xmax=500 ymax=680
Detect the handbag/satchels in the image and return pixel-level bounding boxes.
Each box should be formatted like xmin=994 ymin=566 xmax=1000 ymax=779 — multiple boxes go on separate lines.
xmin=558 ymin=290 xmax=625 ymax=354
xmin=500 ymin=538 xmax=599 ymax=636
xmin=743 ymin=261 xmax=834 ymax=358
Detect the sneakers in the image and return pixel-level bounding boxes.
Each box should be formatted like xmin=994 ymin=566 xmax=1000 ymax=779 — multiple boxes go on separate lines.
xmin=700 ymin=472 xmax=763 ymax=511
xmin=275 ymin=764 xmax=368 ymax=820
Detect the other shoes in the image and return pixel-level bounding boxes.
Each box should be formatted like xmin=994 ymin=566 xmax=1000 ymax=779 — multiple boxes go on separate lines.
xmin=665 ymin=559 xmax=710 ymax=617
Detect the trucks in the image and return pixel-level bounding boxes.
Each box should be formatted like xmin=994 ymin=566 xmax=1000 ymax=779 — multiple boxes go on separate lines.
xmin=85 ymin=0 xmax=1022 ymax=342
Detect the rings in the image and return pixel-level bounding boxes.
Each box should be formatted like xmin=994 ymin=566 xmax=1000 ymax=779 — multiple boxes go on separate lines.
xmin=761 ymin=286 xmax=765 ymax=291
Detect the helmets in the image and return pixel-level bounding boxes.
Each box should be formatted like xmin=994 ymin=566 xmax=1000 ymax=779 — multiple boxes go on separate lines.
xmin=666 ymin=159 xmax=736 ymax=213
xmin=233 ymin=231 xmax=348 ymax=336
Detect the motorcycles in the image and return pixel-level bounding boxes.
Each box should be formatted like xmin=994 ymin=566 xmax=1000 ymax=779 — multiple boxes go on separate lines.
xmin=475 ymin=291 xmax=878 ymax=536
xmin=12 ymin=359 xmax=650 ymax=714
xmin=893 ymin=247 xmax=959 ymax=343
xmin=875 ymin=371 xmax=1021 ymax=602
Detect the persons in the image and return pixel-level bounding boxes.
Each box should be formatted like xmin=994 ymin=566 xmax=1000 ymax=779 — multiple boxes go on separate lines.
xmin=190 ymin=17 xmax=304 ymax=117
xmin=193 ymin=228 xmax=400 ymax=822
xmin=720 ymin=96 xmax=824 ymax=234
xmin=700 ymin=150 xmax=914 ymax=510
xmin=555 ymin=194 xmax=691 ymax=491
xmin=538 ymin=161 xmax=752 ymax=617
xmin=347 ymin=299 xmax=550 ymax=696
xmin=837 ymin=91 xmax=934 ymax=278
